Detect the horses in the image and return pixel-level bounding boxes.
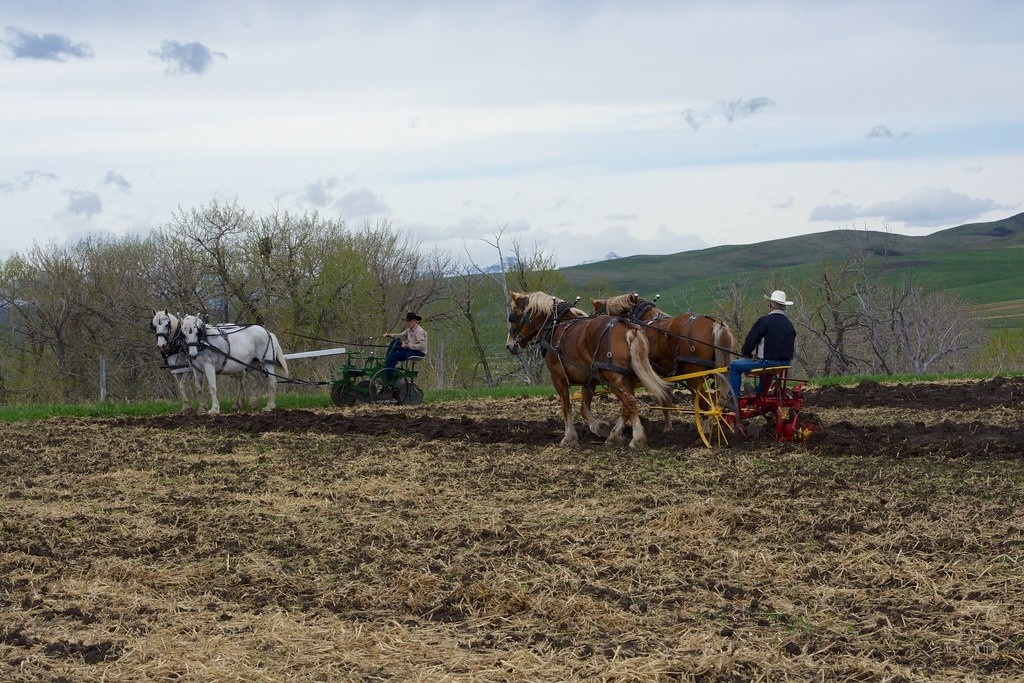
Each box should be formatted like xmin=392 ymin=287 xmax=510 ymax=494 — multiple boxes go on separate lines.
xmin=504 ymin=290 xmax=737 ymax=449
xmin=150 ymin=307 xmax=289 ymax=416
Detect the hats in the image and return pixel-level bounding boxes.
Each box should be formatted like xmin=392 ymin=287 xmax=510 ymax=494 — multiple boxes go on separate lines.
xmin=402 ymin=312 xmax=422 ymax=321
xmin=764 ymin=289 xmax=794 ymax=306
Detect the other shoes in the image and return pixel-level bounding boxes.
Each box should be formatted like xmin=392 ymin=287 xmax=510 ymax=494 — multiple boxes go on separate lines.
xmin=744 ymin=382 xmax=755 ymax=395
xmin=718 ymin=397 xmax=736 ymax=411
xmin=378 ymin=359 xmax=384 ymax=367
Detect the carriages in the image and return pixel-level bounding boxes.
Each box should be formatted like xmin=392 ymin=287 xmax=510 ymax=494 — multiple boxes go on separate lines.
xmin=506 ymin=292 xmax=828 ymax=451
xmin=150 ymin=308 xmax=425 ymax=415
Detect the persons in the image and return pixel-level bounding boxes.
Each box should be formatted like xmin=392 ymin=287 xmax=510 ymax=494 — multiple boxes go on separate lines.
xmin=727 ymin=289 xmax=797 ymax=397
xmin=383 ymin=312 xmax=428 ymax=373
